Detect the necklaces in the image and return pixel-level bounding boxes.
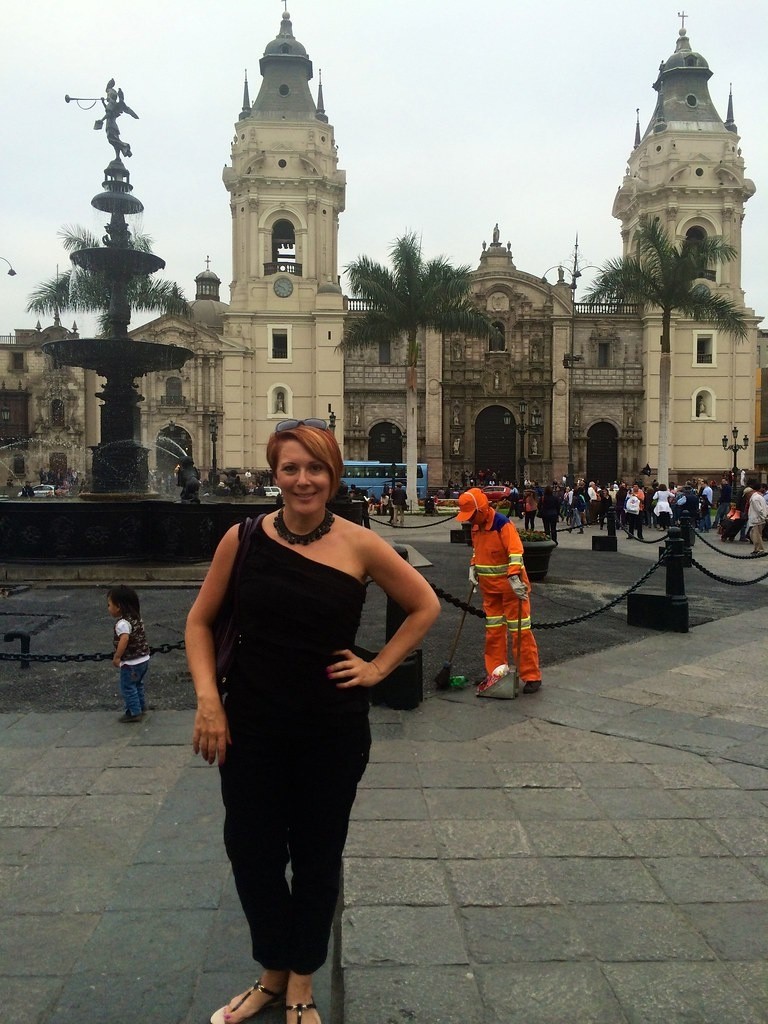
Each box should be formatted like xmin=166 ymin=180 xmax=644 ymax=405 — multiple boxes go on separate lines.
xmin=274 ymin=507 xmax=335 ymax=545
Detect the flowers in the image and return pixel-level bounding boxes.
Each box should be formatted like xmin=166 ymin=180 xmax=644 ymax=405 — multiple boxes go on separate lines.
xmin=516 ymin=528 xmax=552 ymax=542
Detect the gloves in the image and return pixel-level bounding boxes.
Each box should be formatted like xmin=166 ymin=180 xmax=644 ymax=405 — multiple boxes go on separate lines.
xmin=468 ymin=566 xmax=479 ymax=593
xmin=507 ymin=575 xmax=529 ymax=601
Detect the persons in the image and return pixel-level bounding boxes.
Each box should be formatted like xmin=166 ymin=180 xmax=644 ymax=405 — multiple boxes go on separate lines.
xmin=454 ymin=487 xmax=542 ymax=695
xmin=7 ymin=468 xmax=86 ymax=498
xmin=147 ymin=464 xmax=275 ymax=498
xmin=183 ymin=419 xmax=441 ymax=1024
xmin=174 ymin=456 xmax=201 ymax=505
xmin=98 ymin=90 xmax=132 ymax=160
xmin=424 ymin=469 xmax=768 ymax=557
xmin=340 ymin=481 xmax=407 ymax=528
xmin=107 ymin=585 xmax=151 ymax=722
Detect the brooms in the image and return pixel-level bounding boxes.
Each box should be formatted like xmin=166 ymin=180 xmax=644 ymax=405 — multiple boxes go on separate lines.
xmin=433 ymin=577 xmax=478 ymax=692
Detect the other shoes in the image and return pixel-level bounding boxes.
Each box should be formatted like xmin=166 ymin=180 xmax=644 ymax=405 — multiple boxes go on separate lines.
xmin=658 ymin=527 xmax=664 ymax=531
xmin=750 ymin=551 xmax=764 ymax=556
xmin=637 ymin=536 xmax=644 ymax=540
xmin=626 ymin=536 xmax=634 ymax=539
xmin=566 ymin=528 xmax=572 ymax=533
xmin=118 ymin=714 xmax=142 ymax=723
xmin=523 ymin=681 xmax=541 ymax=694
xmin=577 ymin=528 xmax=584 ymax=534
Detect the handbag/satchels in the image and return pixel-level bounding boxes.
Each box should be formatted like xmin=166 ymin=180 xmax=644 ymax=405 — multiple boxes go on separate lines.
xmin=402 ymin=498 xmax=409 ymax=512
xmin=626 ymin=495 xmax=640 ymax=515
xmin=508 ymin=489 xmax=519 ymax=502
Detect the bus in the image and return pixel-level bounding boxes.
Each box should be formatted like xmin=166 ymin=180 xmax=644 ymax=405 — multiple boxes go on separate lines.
xmin=340 ymin=460 xmax=428 ymax=502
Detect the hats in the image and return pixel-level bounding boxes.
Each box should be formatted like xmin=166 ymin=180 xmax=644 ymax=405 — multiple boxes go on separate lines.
xmin=455 ymin=488 xmax=489 ymax=522
xmin=742 ymin=487 xmax=753 ymax=497
xmin=395 ymin=482 xmax=403 ymax=486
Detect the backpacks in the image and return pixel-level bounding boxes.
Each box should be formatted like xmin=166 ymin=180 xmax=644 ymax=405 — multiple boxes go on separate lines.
xmin=575 ymin=496 xmax=585 ymax=512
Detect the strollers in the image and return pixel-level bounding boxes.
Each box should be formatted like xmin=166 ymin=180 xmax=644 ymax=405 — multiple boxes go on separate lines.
xmin=423 ymin=498 xmax=435 ymax=516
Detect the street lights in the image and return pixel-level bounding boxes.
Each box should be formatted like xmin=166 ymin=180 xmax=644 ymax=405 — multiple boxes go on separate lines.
xmin=380 ymin=425 xmax=407 ymax=491
xmin=541 ymin=261 xmax=609 ymax=490
xmin=209 ymin=410 xmax=218 ymax=487
xmin=722 ymin=426 xmax=749 ymax=494
xmin=503 ymin=399 xmax=542 ymax=511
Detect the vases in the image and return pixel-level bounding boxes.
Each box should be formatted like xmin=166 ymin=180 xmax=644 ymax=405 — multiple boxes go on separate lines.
xmin=522 ymin=540 xmax=557 ymax=583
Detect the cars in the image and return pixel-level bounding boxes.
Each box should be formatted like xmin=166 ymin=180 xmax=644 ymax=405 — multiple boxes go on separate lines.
xmin=263 ymin=486 xmax=281 ymax=496
xmin=483 ymin=486 xmax=511 ymax=501
xmin=17 ymin=484 xmax=56 ymax=498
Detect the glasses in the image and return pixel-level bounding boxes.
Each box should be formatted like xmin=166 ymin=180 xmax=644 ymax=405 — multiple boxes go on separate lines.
xmin=468 ymin=509 xmax=477 ymax=522
xmin=274 ymin=418 xmax=328 ymax=432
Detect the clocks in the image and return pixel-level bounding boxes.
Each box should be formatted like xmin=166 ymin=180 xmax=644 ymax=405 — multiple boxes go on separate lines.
xmin=273 ymin=277 xmax=294 ymax=298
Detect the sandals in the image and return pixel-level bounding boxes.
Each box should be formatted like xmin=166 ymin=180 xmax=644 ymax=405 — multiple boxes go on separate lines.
xmin=210 ymin=979 xmax=276 ymax=1024
xmin=286 ymin=992 xmax=321 ymax=1024
